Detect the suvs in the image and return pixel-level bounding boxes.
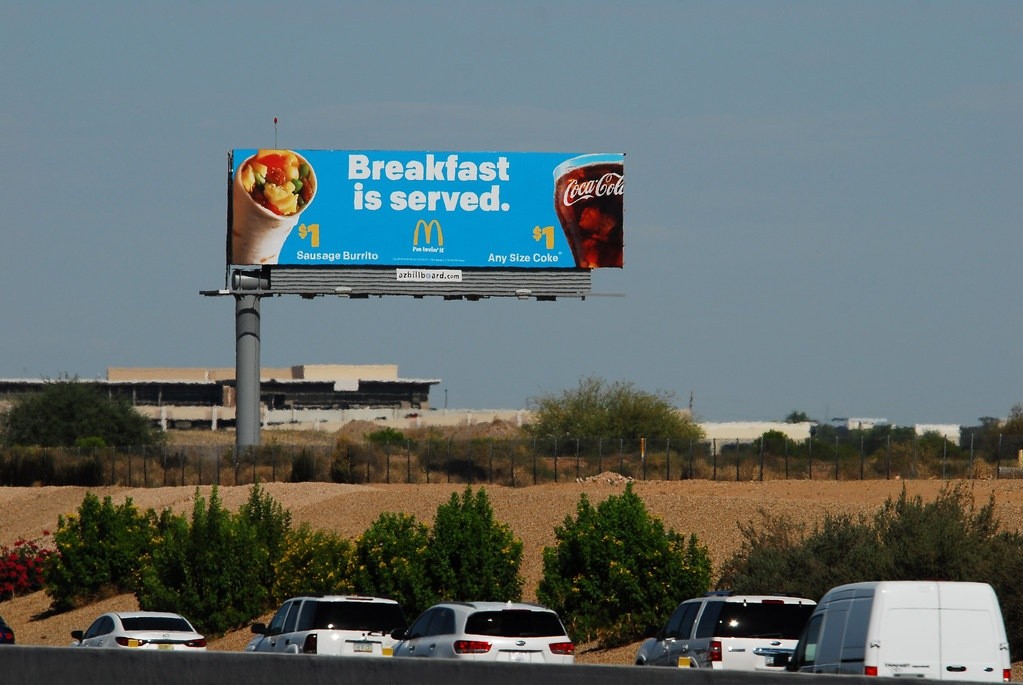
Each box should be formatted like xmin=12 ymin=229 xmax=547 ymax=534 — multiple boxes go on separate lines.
xmin=244 ymin=596 xmax=409 ymax=658
xmin=635 ymin=589 xmax=818 ymax=673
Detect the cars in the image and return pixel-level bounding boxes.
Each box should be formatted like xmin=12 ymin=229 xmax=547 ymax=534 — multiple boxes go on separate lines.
xmin=391 ymin=601 xmax=576 ymax=665
xmin=67 ymin=612 xmax=207 ymax=652
xmin=0 ymin=615 xmax=16 ymax=644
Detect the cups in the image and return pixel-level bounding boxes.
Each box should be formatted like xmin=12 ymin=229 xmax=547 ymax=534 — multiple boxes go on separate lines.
xmin=552 ymin=153 xmax=624 ymax=268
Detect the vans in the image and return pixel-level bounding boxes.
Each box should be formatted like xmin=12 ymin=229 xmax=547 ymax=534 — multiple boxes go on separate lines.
xmin=783 ymin=580 xmax=1014 ymax=683
